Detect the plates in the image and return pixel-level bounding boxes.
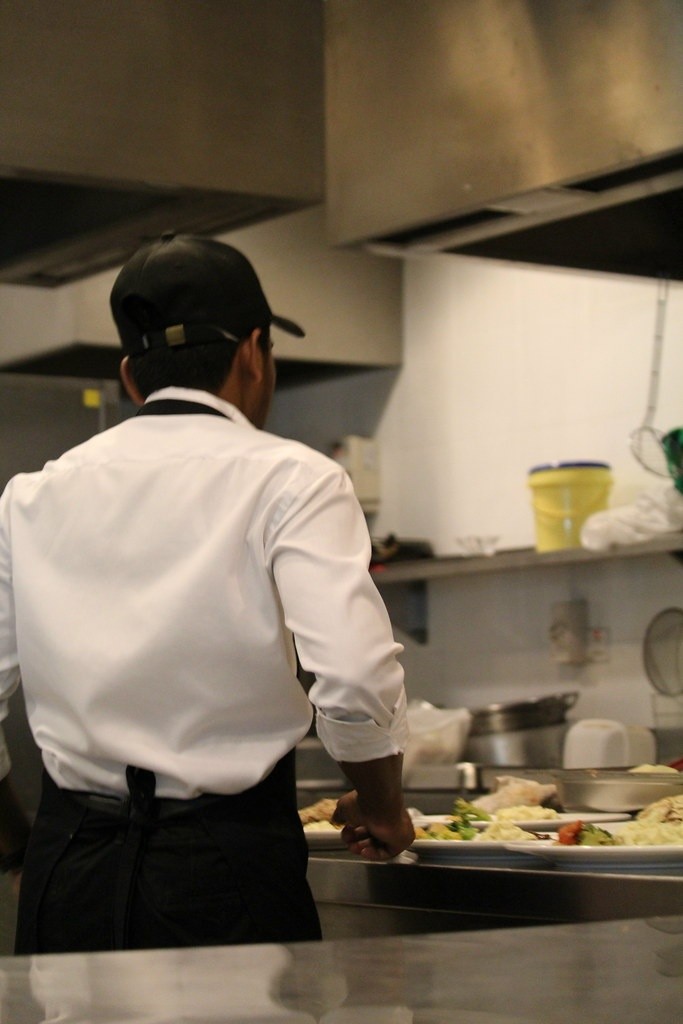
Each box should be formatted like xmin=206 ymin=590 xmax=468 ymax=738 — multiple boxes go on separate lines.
xmin=463 ymin=810 xmax=631 ymax=831
xmin=302 ymin=811 xmax=455 ymax=844
xmin=547 ymin=819 xmax=636 ymax=841
xmin=505 ymin=841 xmax=683 ymax=868
xmin=404 ymin=826 xmax=537 ymax=852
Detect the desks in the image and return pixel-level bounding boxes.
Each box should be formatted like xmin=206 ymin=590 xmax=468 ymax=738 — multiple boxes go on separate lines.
xmin=0 ymin=913 xmax=683 ymax=1024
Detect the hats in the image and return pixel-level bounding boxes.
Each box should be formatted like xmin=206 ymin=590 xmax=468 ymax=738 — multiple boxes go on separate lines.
xmin=110 ymin=229 xmax=306 ymax=354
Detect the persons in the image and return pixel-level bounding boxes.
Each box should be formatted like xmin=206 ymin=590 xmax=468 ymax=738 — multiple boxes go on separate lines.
xmin=1 ymin=228 xmax=416 ymax=955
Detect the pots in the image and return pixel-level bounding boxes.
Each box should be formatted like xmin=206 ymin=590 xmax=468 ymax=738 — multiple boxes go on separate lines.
xmin=437 ymin=686 xmax=580 ymax=739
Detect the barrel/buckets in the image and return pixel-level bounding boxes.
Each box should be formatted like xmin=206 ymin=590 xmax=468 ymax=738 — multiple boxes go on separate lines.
xmin=524 ymin=460 xmax=614 ymax=554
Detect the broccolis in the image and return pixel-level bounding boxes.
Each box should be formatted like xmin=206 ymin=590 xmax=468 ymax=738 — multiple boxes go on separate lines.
xmin=578 ymin=825 xmax=612 ymax=846
xmin=414 ymin=798 xmax=490 ymax=840
